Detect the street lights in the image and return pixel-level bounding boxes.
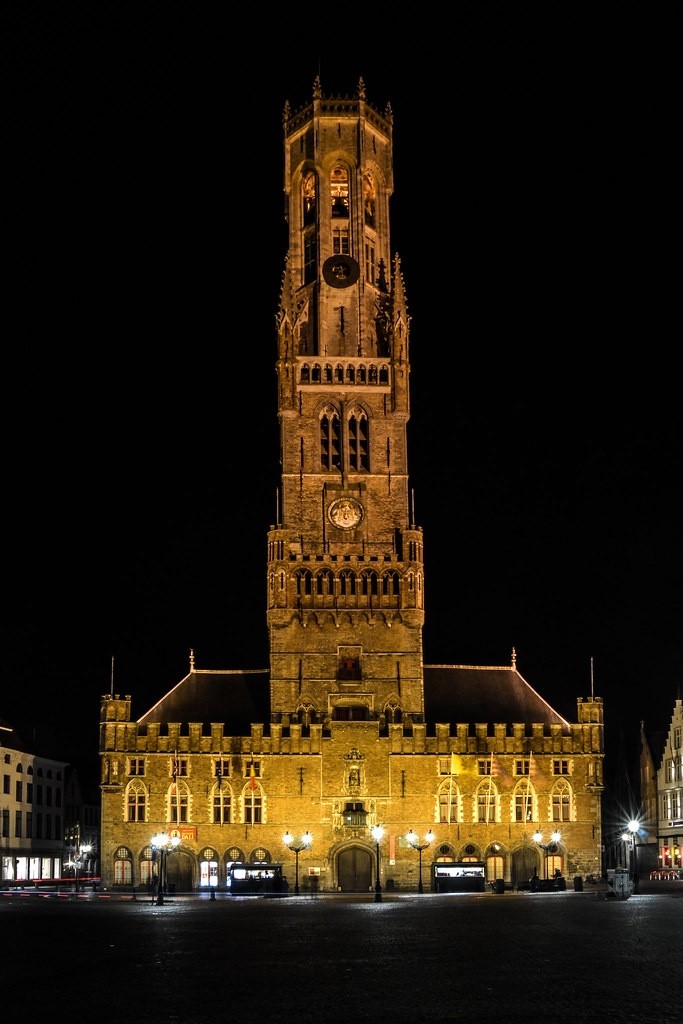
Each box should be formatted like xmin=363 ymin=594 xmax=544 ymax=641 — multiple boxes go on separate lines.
xmin=406 ymin=833 xmax=436 ymax=895
xmin=150 ymin=832 xmax=179 ymax=906
xmin=373 ymin=826 xmax=389 ymax=904
xmin=533 ymin=831 xmax=561 ymax=879
xmin=628 ymin=819 xmax=641 ymax=892
xmin=284 ymin=833 xmax=310 ymax=897
xmin=80 ymin=845 xmax=92 ymax=875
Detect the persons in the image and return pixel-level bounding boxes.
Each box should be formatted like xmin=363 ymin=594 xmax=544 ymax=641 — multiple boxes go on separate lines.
xmin=153 ymin=872 xmax=159 ymax=892
xmin=553 ymin=868 xmax=561 ymax=879
xmin=245 ymin=871 xmax=272 ymax=879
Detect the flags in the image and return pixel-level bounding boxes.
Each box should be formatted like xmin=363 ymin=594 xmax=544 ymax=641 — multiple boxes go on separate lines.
xmin=529 ymin=756 xmax=553 ymax=791
xmin=171 ymin=759 xmax=177 ymax=793
xmin=492 ymin=755 xmax=514 ymax=785
xmin=249 ymin=758 xmax=256 ymax=791
xmin=213 ymin=758 xmax=222 ymax=790
xmin=451 ymin=755 xmax=476 ymax=776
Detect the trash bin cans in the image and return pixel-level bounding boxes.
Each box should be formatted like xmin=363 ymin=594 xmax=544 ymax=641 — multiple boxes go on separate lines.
xmin=496 ymin=879 xmax=504 ymax=894
xmin=574 ymin=877 xmax=582 ymax=892
